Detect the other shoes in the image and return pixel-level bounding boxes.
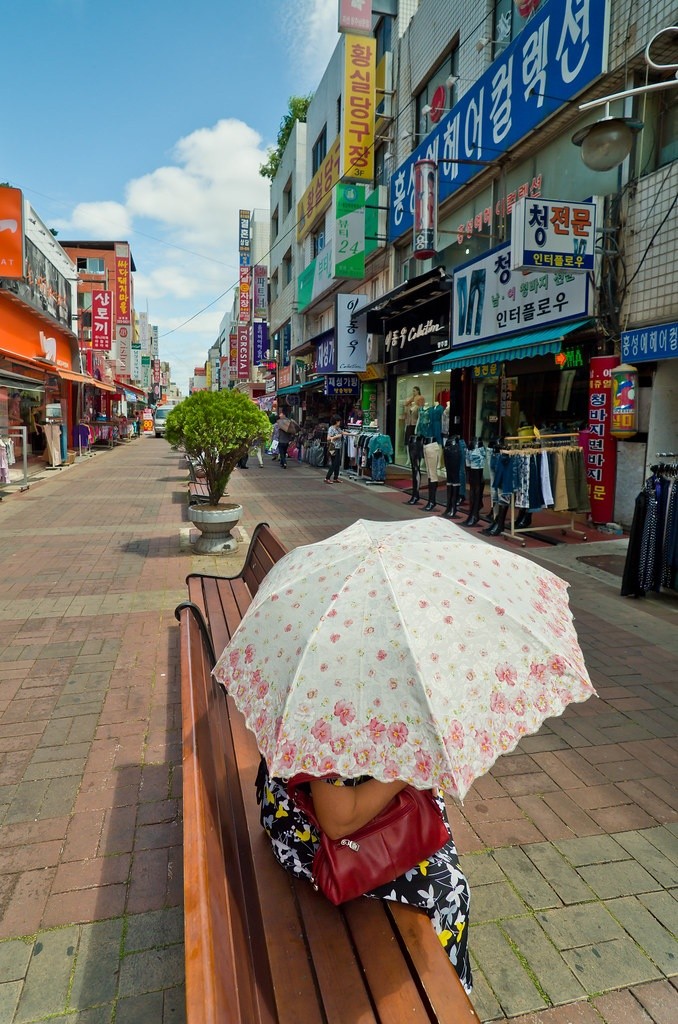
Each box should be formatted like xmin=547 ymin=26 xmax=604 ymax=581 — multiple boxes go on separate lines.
xmin=241 ymin=466 xmax=248 ymax=469
xmin=280 ymin=462 xmax=287 ymax=468
xmin=260 ymin=463 xmax=264 ymax=467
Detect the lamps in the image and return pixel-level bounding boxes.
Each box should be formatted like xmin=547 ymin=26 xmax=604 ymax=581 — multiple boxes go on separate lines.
xmin=572 ymin=25 xmax=678 ymax=172
xmin=383 ymin=38 xmax=512 ymax=161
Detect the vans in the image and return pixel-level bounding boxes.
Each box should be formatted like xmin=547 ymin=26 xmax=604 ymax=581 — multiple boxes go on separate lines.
xmin=154 ymin=405 xmax=175 ymax=437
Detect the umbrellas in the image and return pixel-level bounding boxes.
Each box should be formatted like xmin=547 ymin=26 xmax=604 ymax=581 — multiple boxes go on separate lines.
xmin=211 ymin=515 xmax=601 ymax=804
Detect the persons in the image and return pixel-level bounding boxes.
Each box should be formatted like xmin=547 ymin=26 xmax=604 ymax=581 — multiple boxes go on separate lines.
xmin=271 ymin=412 xmax=293 ymax=468
xmin=8 ymin=393 xmax=24 ymax=459
xmin=404 ymin=433 xmax=429 ymax=505
xmin=405 ymin=385 xmax=425 ymax=410
xmin=403 ymin=401 xmax=456 ymax=469
xmin=253 ymin=751 xmax=474 ymax=995
xmin=323 ymin=414 xmax=356 ymax=484
xmin=236 ymin=452 xmax=249 ymax=469
xmin=457 ymin=437 xmax=487 ymax=526
xmin=480 ymin=444 xmax=532 ymax=537
xmin=422 ymin=437 xmax=447 ymax=512
xmin=254 ymin=439 xmax=266 ymax=468
xmin=440 ymin=435 xmax=469 ymax=519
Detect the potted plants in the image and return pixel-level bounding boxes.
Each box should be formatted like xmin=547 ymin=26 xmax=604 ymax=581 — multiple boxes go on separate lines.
xmin=167 ymin=388 xmax=275 ymax=557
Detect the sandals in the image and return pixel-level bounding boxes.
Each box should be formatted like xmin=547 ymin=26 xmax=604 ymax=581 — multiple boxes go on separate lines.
xmin=324 ymin=478 xmax=333 ymax=484
xmin=333 ymin=479 xmax=343 ymax=483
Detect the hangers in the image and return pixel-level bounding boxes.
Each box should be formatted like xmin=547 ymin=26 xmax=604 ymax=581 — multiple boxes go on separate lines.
xmin=650 ymin=452 xmax=678 ymax=480
xmin=348 ymin=429 xmax=381 ymax=438
xmin=499 ymin=442 xmax=584 ymax=455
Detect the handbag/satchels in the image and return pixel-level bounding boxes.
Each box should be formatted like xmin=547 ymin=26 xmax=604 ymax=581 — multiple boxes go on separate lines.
xmin=288 ymin=773 xmax=450 ymax=905
xmin=327 ymin=440 xmax=336 ymax=456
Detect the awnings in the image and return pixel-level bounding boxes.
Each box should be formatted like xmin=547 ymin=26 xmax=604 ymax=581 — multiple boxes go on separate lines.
xmin=433 ymin=319 xmax=594 ymax=373
xmin=91 ymin=376 xmax=118 ymax=393
xmin=351 ymin=265 xmax=453 ymax=321
xmin=0 ymin=368 xmax=45 ymax=394
xmin=114 ymin=379 xmax=150 ymax=399
xmin=288 ymin=326 xmax=334 ymax=357
xmin=307 ymin=371 xmax=356 ymax=379
xmin=56 ymin=367 xmax=94 ymax=388
xmin=275 ymin=377 xmax=325 ymax=397
xmin=257 ymin=390 xmax=285 ymax=412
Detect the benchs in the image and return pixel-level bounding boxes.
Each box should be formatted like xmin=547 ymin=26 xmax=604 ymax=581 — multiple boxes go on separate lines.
xmin=174 ymin=602 xmax=485 ymax=1024
xmin=184 ymin=454 xmax=230 ymax=506
xmin=186 ymin=521 xmax=288 ymax=664
